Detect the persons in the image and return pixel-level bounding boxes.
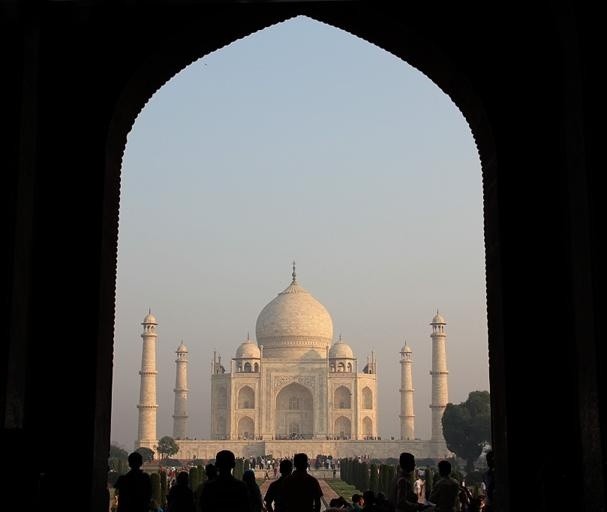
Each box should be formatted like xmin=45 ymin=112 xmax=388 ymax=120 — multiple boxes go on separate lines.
xmin=108 ymin=451 xmax=493 ymax=511
